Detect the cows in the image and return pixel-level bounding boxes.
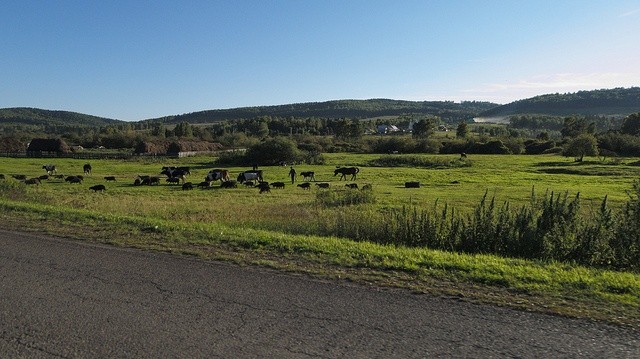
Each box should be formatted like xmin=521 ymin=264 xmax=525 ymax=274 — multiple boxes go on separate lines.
xmin=220 ymin=180 xmax=238 ymax=189
xmin=460 ymin=153 xmax=467 ymax=158
xmin=208 ymin=168 xmax=231 ymax=181
xmin=296 ymin=182 xmax=312 ymax=190
xmin=181 ymin=182 xmax=194 ymax=191
xmin=176 ymin=166 xmax=192 ymax=176
xmin=42 ymin=165 xmax=58 ymax=175
xmin=344 ymin=183 xmax=359 ymax=190
xmin=103 ymin=176 xmax=117 ymax=182
xmin=315 ymin=182 xmax=329 ymax=190
xmin=65 ymin=175 xmax=77 ymax=182
xmin=137 ymin=174 xmax=151 ymax=182
xmin=166 ymin=177 xmax=180 ymax=185
xmin=54 ymin=174 xmax=64 ymax=180
xmin=273 ymin=183 xmax=285 ymax=189
xmin=269 ymin=181 xmax=281 ymax=187
xmin=254 ymin=184 xmax=266 ymax=189
xmin=197 ymin=181 xmax=210 ymax=189
xmin=134 ymin=178 xmax=141 ymax=186
xmin=161 ymin=165 xmax=177 ymax=171
xmin=160 ymin=169 xmax=188 ymax=183
xmin=141 ymin=176 xmax=161 ymax=186
xmin=204 ymin=172 xmax=225 ymax=183
xmin=25 ymin=177 xmax=42 ymax=185
xmin=244 ymin=169 xmax=264 ymax=181
xmin=333 ymin=166 xmax=360 ymax=181
xmin=236 ymin=172 xmax=260 ymax=184
xmin=258 ymin=186 xmax=271 ymax=194
xmin=38 ymin=174 xmax=49 ymax=180
xmin=83 ymin=163 xmax=92 ymax=174
xmin=12 ymin=174 xmax=27 ymax=181
xmin=88 ymin=184 xmax=106 ymax=193
xmin=299 ymin=171 xmax=316 ymax=182
xmin=75 ymin=175 xmax=84 ymax=182
xmin=70 ymin=178 xmax=82 ymax=185
xmin=243 ymin=181 xmax=254 ymax=187
xmin=258 ymin=181 xmax=268 ymax=185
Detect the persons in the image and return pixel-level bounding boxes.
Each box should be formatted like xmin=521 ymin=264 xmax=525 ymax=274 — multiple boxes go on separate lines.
xmin=289 ymin=166 xmax=296 ymax=186
